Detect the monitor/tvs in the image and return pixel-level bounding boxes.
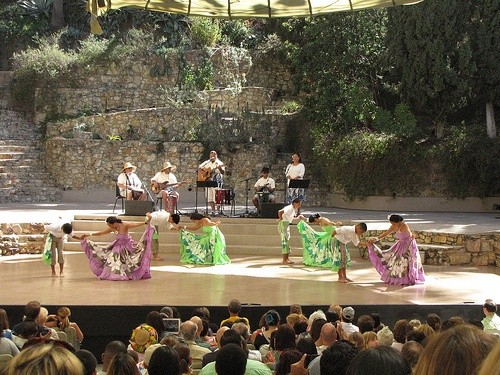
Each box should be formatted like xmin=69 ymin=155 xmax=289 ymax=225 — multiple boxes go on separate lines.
xmin=163 ymin=318 xmax=180 ymax=334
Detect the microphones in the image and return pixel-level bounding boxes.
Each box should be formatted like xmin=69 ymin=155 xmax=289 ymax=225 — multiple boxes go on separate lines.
xmin=210 ymin=157 xmax=215 ymax=161
xmin=241 ymin=176 xmax=255 ymax=182
xmin=124 ymin=172 xmax=131 ymax=186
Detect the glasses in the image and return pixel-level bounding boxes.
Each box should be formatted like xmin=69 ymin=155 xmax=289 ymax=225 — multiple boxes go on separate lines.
xmin=22 ymin=339 xmax=76 ymax=355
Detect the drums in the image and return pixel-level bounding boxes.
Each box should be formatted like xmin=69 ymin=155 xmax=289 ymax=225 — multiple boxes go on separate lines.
xmin=215 ymin=189 xmax=231 ymax=206
xmin=256 ymin=192 xmax=270 ymax=212
xmin=132 ymin=190 xmax=143 ymax=200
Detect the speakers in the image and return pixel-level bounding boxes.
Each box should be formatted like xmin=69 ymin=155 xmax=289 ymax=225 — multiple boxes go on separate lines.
xmin=125 ymin=200 xmax=154 ymax=216
xmin=257 ymin=203 xmax=287 ymax=218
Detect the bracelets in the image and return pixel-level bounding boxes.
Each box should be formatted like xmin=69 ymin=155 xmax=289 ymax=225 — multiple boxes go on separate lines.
xmin=90 ymin=234 xmax=92 ymax=236
xmin=184 ymin=226 xmax=186 ymax=229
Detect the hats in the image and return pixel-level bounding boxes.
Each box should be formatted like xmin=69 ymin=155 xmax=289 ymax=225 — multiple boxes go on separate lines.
xmin=161 ymin=162 xmax=173 ymax=171
xmin=343 ymin=307 xmax=355 ymax=319
xmin=144 ymin=344 xmax=167 ymax=366
xmin=121 ymin=163 xmax=136 ymax=172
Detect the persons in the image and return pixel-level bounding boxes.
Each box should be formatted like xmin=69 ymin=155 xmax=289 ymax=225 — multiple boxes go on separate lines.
xmin=117 ymin=163 xmax=148 ymax=200
xmin=285 ymin=153 xmax=306 ymax=203
xmin=297 ymin=213 xmax=351 ymax=268
xmin=200 ymin=151 xmax=225 ymax=213
xmin=0 ymin=299 xmax=500 ymax=375
xmin=276 ymin=198 xmax=303 ymax=264
xmin=252 ymin=167 xmax=276 ymax=209
xmin=145 ymin=210 xmax=180 ymax=260
xmin=151 ymin=162 xmax=179 ymax=212
xmin=47 ymin=222 xmax=84 ymax=277
xmin=180 ymin=212 xmax=231 ymax=265
xmin=81 ymin=213 xmax=155 ymax=280
xmin=330 ymin=222 xmax=369 ymax=282
xmin=368 ymin=214 xmax=425 ymax=286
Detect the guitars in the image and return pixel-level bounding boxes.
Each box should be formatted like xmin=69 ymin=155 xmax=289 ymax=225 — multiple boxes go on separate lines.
xmin=198 ymin=163 xmax=227 ymax=181
xmin=151 ymin=178 xmax=192 ymax=194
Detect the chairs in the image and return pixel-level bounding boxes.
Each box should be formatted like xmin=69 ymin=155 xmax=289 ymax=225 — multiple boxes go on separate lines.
xmin=223 ymin=185 xmax=236 ymax=214
xmin=113 ymin=183 xmax=124 ymax=214
xmin=155 ymin=191 xmax=178 ymax=212
